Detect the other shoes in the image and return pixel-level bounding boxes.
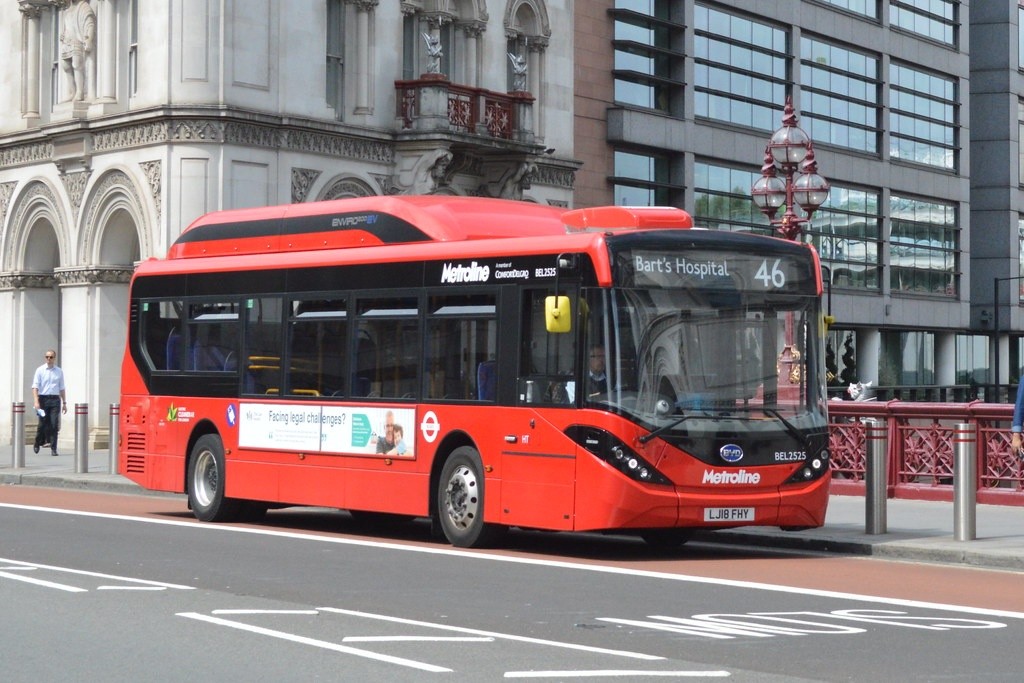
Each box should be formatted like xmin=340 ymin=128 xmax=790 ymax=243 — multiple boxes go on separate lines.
xmin=34 ymin=442 xmax=39 ymax=453
xmin=52 ymin=450 xmax=58 ymax=456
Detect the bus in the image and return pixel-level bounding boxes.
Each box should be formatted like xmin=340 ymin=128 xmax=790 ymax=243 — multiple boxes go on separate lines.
xmin=116 ymin=194 xmax=836 ymax=553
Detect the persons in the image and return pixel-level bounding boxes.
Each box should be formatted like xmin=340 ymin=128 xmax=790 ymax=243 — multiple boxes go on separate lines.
xmin=735 ymin=314 xmax=761 ymax=399
xmin=376 ymin=410 xmax=411 ymax=456
xmin=31 ymin=350 xmax=67 ymax=456
xmin=425 ymin=148 xmax=453 ymax=178
xmin=60 ymin=0 xmax=96 ymax=103
xmin=565 ymin=342 xmax=613 ymax=405
xmin=1012 ymin=373 xmax=1024 ymax=459
xmin=512 ymin=162 xmax=539 ymax=190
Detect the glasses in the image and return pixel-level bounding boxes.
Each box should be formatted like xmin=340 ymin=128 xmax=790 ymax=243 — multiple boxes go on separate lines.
xmin=45 ymin=355 xmax=53 ymax=359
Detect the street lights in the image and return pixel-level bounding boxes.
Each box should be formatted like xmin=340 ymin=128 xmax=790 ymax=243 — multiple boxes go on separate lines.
xmin=749 ymin=95 xmax=833 ymax=407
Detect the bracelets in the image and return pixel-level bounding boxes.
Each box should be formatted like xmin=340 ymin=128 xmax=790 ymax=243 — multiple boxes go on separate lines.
xmin=63 ymin=401 xmax=67 ymax=404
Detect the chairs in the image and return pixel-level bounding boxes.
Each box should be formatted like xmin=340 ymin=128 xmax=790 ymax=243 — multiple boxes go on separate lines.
xmin=163 ymin=326 xmax=495 ymax=401
